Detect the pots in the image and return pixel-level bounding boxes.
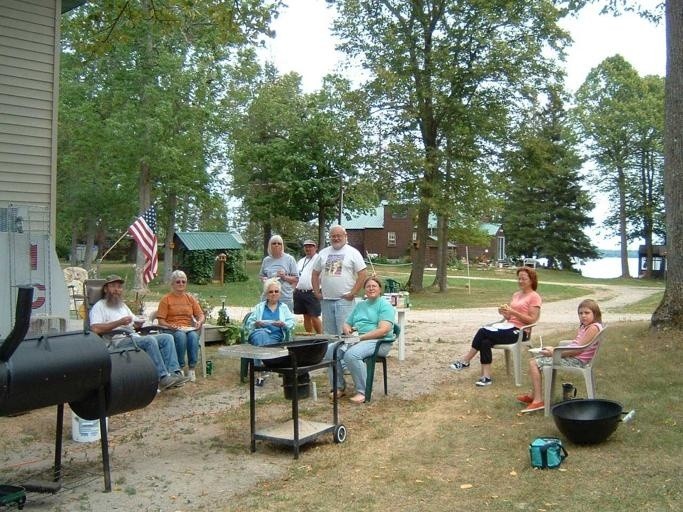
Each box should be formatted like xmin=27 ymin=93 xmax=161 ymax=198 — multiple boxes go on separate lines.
xmin=551 ymin=398 xmax=629 ymax=444
xmin=258 ymin=338 xmax=328 ymax=368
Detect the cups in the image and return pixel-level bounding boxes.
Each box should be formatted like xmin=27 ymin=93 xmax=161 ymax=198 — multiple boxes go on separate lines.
xmin=151 ymin=319 xmax=158 ymax=325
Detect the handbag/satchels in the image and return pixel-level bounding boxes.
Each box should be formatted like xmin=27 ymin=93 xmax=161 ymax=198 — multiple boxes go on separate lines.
xmin=529 ymin=436 xmax=562 ymax=468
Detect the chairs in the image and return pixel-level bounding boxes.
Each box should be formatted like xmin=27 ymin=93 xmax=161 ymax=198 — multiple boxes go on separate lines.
xmin=329 ymin=319 xmax=401 ymax=401
xmin=148 ymin=308 xmax=208 ymax=382
xmin=79 ymin=279 xmax=168 ymax=391
xmin=239 ymin=310 xmax=298 ymax=385
xmin=529 ymin=325 xmax=606 ymax=417
xmin=477 ymin=313 xmax=549 ymax=387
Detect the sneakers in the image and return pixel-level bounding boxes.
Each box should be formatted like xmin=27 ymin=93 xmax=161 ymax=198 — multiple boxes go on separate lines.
xmin=159 ymin=369 xmax=197 ymax=389
xmin=475 ymin=376 xmax=492 ymax=386
xmin=516 ymin=395 xmax=533 ymax=404
xmin=349 ymin=393 xmax=365 ymax=404
xmin=521 ymin=401 xmax=544 ymax=413
xmin=449 ymin=361 xmax=470 ymax=371
xmin=255 ymin=377 xmax=264 ymax=388
xmin=330 ymin=391 xmax=344 ymax=399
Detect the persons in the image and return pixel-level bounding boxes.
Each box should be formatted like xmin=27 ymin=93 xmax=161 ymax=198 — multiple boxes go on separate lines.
xmin=513 ymin=298 xmax=604 ymax=416
xmin=88 ymin=272 xmax=193 ymax=394
xmin=310 ymin=223 xmax=369 ymax=377
xmin=243 ymin=278 xmax=295 ymax=388
xmin=447 ymin=267 xmax=544 ymax=389
xmin=259 ymin=233 xmax=300 ymax=340
xmin=323 ymin=274 xmax=397 ymax=406
xmin=155 ymin=267 xmax=207 ymax=383
xmin=292 ymin=238 xmax=322 ymax=335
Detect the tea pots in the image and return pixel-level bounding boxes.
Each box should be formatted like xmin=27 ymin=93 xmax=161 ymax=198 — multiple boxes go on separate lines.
xmin=562 ymin=382 xmax=576 ymax=401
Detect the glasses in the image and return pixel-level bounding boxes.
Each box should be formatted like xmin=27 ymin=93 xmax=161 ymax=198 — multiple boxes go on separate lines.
xmin=176 ymin=280 xmax=186 ymax=284
xmin=272 ymin=243 xmax=282 ymax=245
xmin=269 ymin=290 xmax=279 ymax=293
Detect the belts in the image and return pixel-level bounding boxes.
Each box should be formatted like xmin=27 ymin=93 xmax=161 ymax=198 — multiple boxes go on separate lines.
xmin=298 ymin=289 xmax=312 ymax=293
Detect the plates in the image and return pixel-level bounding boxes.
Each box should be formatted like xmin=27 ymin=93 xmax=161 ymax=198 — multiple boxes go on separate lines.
xmin=260 ymin=320 xmax=275 ymax=323
xmin=133 ymin=318 xmax=146 ymax=322
xmin=492 ymin=322 xmax=514 ymax=330
xmin=527 ymin=347 xmax=542 ymax=354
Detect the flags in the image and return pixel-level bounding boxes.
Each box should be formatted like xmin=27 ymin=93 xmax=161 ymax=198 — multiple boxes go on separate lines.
xmin=127 ymin=203 xmax=160 ymax=287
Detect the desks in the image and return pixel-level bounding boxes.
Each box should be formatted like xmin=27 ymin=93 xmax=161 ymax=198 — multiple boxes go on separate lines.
xmin=392 ymin=303 xmax=412 ymax=362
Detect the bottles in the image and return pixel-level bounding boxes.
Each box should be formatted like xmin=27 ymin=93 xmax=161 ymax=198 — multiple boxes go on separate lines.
xmin=205 ymin=360 xmax=211 ymax=376
xmin=383 ymin=289 xmax=409 ymax=308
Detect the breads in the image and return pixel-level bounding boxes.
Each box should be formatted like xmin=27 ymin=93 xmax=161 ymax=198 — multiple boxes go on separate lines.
xmin=500 ymin=304 xmax=507 ymax=308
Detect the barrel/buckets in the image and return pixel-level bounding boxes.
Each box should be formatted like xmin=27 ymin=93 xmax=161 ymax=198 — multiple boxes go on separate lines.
xmin=70 ymin=411 xmax=110 ymax=444
xmin=283 ymin=374 xmax=311 ymax=401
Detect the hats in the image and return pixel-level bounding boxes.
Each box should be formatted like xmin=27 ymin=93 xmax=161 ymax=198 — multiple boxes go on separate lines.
xmin=304 ymin=240 xmax=316 ymax=245
xmin=104 ymin=275 xmax=125 ymax=283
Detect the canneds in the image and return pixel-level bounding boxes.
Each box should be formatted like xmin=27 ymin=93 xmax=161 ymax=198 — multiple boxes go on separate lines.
xmin=206 ymin=361 xmax=212 ymax=376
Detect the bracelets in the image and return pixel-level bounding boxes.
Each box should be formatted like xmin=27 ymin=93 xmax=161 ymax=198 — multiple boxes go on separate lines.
xmin=199 ymin=320 xmax=203 ymax=326
